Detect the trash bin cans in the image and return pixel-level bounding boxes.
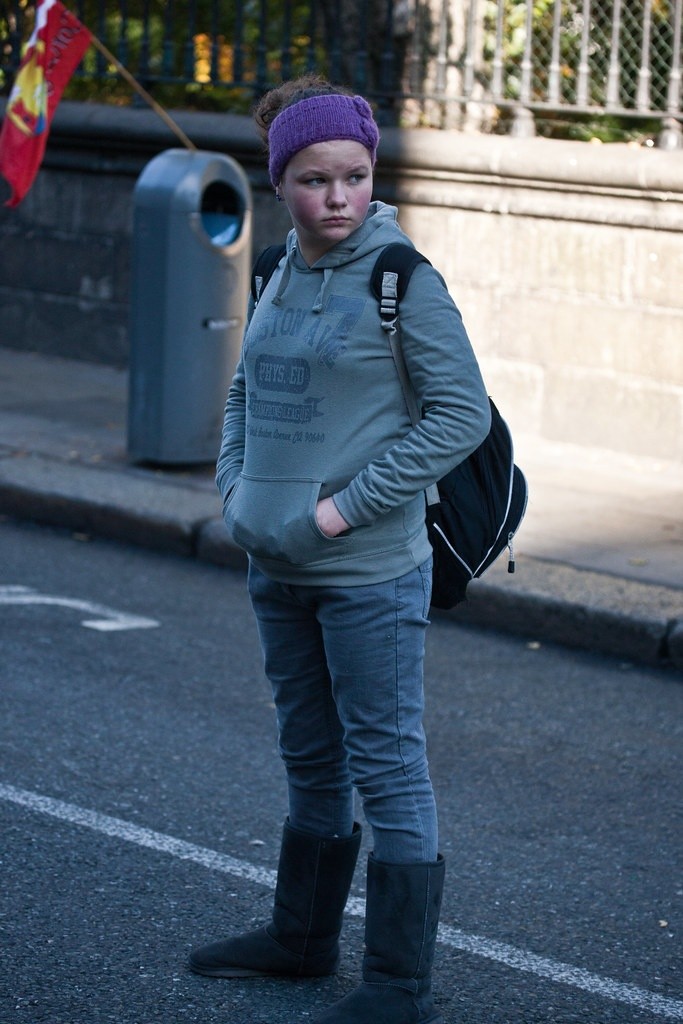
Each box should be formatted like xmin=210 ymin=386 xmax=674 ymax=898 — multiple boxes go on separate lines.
xmin=124 ymin=147 xmax=257 ymax=471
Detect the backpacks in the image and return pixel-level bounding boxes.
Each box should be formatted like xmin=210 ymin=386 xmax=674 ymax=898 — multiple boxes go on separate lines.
xmin=248 ymin=242 xmax=527 ymax=610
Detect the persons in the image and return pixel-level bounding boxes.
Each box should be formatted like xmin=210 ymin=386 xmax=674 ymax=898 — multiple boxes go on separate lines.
xmin=186 ymin=74 xmax=491 ymax=1024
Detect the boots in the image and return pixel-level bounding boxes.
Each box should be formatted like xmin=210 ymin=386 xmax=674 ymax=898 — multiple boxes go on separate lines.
xmin=188 ymin=816 xmax=363 ymax=978
xmin=312 ymin=852 xmax=445 ymax=1024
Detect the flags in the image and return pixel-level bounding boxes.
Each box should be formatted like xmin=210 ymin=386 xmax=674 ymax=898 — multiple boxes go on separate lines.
xmin=0 ymin=0 xmax=92 ymax=208
